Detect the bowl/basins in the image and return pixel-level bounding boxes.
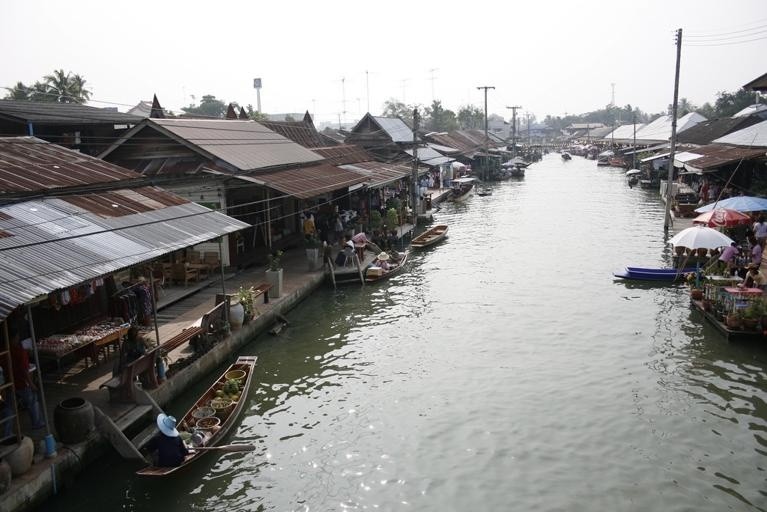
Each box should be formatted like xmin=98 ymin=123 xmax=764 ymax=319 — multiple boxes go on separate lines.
xmin=225 ymin=370 xmax=246 ymax=385
xmin=191 ymin=406 xmax=216 ymax=418
xmin=211 ymin=398 xmax=232 ymax=417
xmin=195 ymin=417 xmax=220 ymax=428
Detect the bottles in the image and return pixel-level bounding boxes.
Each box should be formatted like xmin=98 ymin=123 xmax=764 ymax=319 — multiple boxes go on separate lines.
xmin=735 ymin=271 xmax=738 ymax=279
xmin=734 ymin=251 xmax=750 ymax=266
xmin=43 ymin=433 xmax=55 ymax=455
xmin=156 ymin=355 xmax=165 ymax=379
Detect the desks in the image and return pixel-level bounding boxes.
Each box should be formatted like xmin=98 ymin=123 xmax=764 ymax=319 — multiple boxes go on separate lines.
xmin=33 ymin=318 xmax=132 ymax=386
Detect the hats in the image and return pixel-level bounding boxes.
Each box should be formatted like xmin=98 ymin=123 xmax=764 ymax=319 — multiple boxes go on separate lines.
xmin=377 ymin=251 xmax=390 ymax=260
xmin=744 ymin=263 xmax=760 ymax=269
xmin=156 ymin=413 xmax=180 ymax=437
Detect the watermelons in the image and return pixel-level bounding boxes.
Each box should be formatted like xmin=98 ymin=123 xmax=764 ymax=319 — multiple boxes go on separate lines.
xmin=214 ymin=379 xmax=242 ymax=402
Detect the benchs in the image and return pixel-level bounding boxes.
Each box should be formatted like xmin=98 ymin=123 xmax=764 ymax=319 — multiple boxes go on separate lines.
xmin=183 ymin=299 xmax=229 ymax=349
xmin=253 ymin=282 xmax=274 ymax=303
xmin=99 ymin=327 xmax=205 ymax=402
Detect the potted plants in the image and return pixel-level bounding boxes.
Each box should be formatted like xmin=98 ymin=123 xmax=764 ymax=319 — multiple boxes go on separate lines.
xmin=303 ymin=233 xmax=322 ymax=269
xmin=239 ymin=286 xmax=256 ymax=322
xmin=264 ymin=248 xmax=286 ymax=298
xmin=687 ymin=271 xmax=767 ymax=331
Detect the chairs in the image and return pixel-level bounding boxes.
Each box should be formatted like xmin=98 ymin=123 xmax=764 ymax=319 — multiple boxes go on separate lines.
xmin=152 ymin=249 xmax=221 ymax=290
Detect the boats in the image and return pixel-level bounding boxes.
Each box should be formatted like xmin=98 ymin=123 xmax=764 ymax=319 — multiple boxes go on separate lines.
xmin=364 ymin=251 xmax=408 ymax=284
xmin=612 ymin=266 xmax=704 ymax=285
xmin=477 ymin=144 xmax=698 ymax=218
xmin=446 ymin=183 xmax=475 ymax=203
xmin=411 ymin=225 xmax=449 ymax=248
xmin=135 ymin=355 xmax=259 ymax=479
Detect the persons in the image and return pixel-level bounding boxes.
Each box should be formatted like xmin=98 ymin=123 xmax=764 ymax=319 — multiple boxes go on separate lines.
xmin=146 ymin=412 xmax=189 ymax=469
xmin=629 ymin=174 xmax=637 ymax=182
xmin=300 ymin=155 xmax=465 ymax=275
xmin=0 ymin=319 xmax=48 ymax=443
xmin=116 ymin=323 xmax=168 ymax=383
xmin=688 ymin=172 xmax=766 ymax=312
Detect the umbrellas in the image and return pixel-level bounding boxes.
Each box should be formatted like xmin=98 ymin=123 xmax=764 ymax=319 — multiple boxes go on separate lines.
xmin=626 ymin=168 xmax=641 ymax=175
xmin=665 ymin=224 xmax=736 ymax=254
